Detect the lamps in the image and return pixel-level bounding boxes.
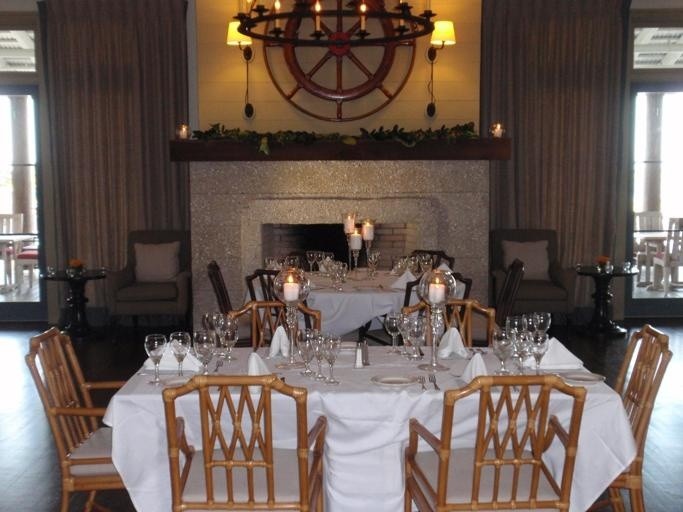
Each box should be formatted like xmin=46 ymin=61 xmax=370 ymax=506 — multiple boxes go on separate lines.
xmin=233 ymin=1 xmax=437 ymax=45
xmin=226 ymin=22 xmax=252 ymax=60
xmin=428 ymin=21 xmax=456 ymax=60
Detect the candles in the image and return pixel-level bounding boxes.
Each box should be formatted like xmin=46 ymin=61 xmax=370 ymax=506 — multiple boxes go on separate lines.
xmin=180 ymin=126 xmax=188 ymax=138
xmin=494 ymin=123 xmax=503 ymax=137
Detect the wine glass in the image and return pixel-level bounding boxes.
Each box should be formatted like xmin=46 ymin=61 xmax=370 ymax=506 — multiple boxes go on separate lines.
xmin=391 ymin=253 xmax=432 ymax=286
xmin=366 ymin=248 xmax=381 ymax=281
xmin=295 ymin=327 xmax=341 ymax=386
xmin=383 ymin=311 xmax=428 ymax=361
xmin=142 ymin=313 xmax=239 ymax=389
xmin=492 ymin=311 xmax=550 ymax=386
xmin=266 ymin=250 xmax=347 ymax=294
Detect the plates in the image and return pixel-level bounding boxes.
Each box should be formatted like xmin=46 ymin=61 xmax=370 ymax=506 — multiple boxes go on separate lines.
xmin=367 ymin=373 xmax=418 ymax=389
xmin=555 ymin=372 xmax=606 ymax=388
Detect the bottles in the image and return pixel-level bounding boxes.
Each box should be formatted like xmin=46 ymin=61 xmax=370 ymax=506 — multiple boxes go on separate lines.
xmin=352 ymin=340 xmax=363 ymax=369
xmin=360 ymin=339 xmax=369 ymax=366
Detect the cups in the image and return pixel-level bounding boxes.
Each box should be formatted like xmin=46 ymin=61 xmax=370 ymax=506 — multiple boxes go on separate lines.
xmin=603 ymin=260 xmax=614 ymax=273
xmin=623 ymin=261 xmax=631 ymax=271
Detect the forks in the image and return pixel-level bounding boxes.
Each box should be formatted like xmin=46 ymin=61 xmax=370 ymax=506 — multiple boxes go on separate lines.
xmin=416 ymin=376 xmax=427 ymax=391
xmin=428 ymin=375 xmax=440 ymax=391
